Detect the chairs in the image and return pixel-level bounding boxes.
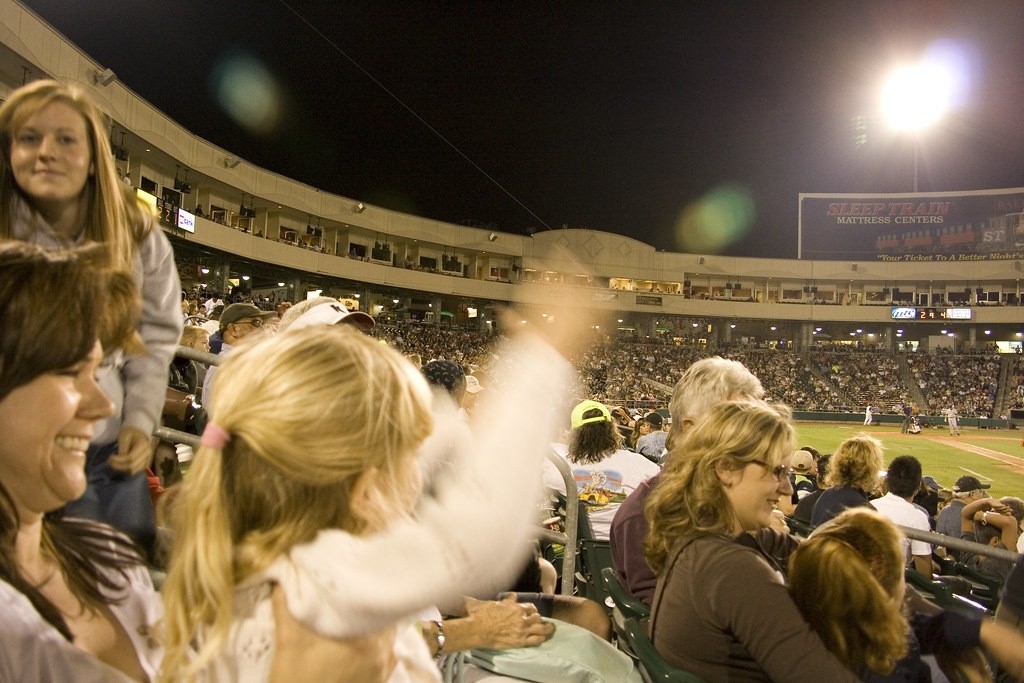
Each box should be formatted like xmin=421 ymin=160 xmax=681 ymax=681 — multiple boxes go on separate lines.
xmin=577 ymin=497 xmax=1004 ymax=683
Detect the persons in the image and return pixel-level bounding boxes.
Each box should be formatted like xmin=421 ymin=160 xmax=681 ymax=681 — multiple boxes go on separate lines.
xmin=0 ymin=79 xmax=1024 ymax=683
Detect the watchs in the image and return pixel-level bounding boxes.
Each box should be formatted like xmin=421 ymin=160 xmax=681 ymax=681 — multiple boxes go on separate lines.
xmin=981 ymin=514 xmax=987 ymax=526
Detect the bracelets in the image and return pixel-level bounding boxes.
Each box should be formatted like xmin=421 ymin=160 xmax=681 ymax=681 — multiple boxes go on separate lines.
xmin=430 ymin=619 xmax=445 ymax=659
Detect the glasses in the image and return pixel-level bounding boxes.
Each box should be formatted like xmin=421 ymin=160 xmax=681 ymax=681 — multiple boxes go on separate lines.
xmin=751 ymin=459 xmax=789 ymax=482
xmin=227 ymin=319 xmax=264 ymax=327
xmin=969 ymin=490 xmax=985 ymax=497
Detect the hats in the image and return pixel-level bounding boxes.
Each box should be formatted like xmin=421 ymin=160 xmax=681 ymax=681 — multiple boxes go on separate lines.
xmin=792 ymin=450 xmax=813 ymax=470
xmin=647 ymin=413 xmax=662 ymax=425
xmin=465 ymin=376 xmax=486 ymax=395
xmin=571 ymin=399 xmax=611 ymax=429
xmin=219 ymin=303 xmax=278 ymax=333
xmin=953 ymin=476 xmax=991 ymax=493
xmin=288 ymin=303 xmax=375 ymax=335
xmin=817 ymin=453 xmax=833 ymax=475
xmin=419 ymin=360 xmax=467 ymax=408
xmin=922 ymin=476 xmax=944 ymax=489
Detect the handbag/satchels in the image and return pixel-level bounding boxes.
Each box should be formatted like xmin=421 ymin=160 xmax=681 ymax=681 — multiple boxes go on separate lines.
xmin=446 ymin=616 xmax=634 ymax=683
